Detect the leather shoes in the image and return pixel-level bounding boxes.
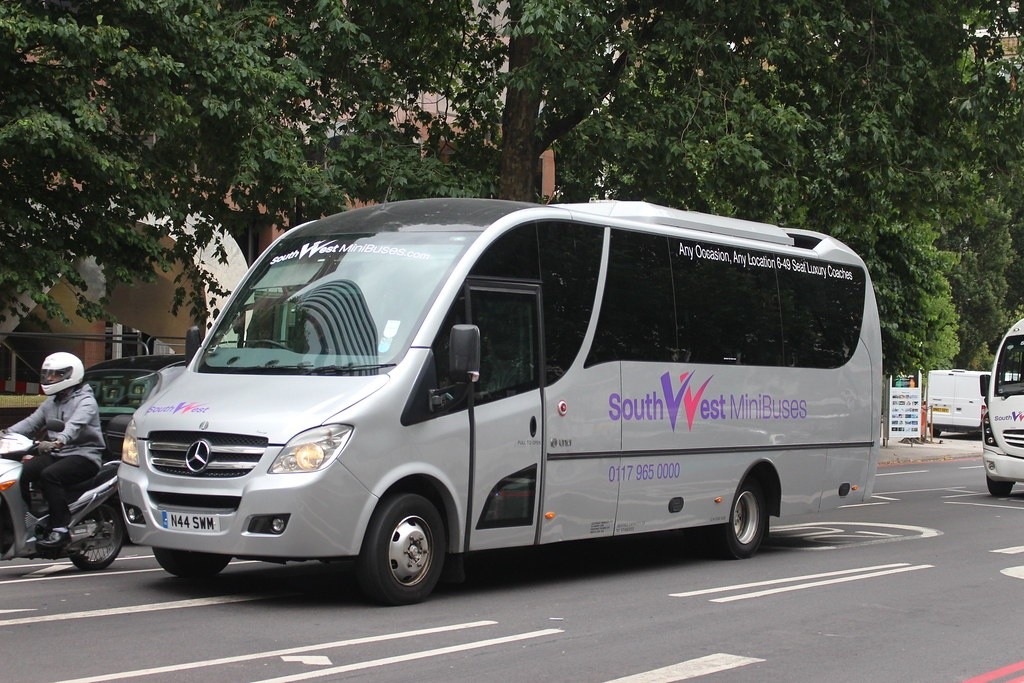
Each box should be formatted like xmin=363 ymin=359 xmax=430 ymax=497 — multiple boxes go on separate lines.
xmin=37 ymin=530 xmax=72 ymax=549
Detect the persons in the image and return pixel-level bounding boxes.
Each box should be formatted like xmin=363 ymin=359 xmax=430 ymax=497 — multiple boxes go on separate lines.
xmin=0 ymin=352 xmax=107 ymax=550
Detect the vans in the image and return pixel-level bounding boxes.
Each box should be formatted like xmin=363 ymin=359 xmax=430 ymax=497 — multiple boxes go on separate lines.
xmin=924 ymin=369 xmax=1022 ymax=438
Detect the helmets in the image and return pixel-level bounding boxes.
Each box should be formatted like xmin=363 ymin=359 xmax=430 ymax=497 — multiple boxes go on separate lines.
xmin=41 ymin=351 xmax=84 ymax=395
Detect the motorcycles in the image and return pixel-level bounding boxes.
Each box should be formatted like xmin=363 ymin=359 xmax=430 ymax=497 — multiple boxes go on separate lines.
xmin=0 ymin=417 xmax=127 ymax=573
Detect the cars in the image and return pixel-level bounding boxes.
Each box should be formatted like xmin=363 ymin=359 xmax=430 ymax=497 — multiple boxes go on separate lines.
xmin=81 ymin=349 xmax=187 ymax=546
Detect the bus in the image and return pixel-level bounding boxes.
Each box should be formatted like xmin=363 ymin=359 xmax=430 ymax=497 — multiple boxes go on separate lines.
xmin=115 ymin=196 xmax=883 ymax=606
xmin=978 ymin=316 xmax=1024 ymax=497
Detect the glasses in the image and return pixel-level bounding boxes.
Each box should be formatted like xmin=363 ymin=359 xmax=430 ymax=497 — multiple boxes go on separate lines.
xmin=48 ymin=370 xmax=70 ymax=377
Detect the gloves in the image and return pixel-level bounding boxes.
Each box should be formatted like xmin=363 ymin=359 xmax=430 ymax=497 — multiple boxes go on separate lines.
xmin=38 ymin=439 xmax=58 ymax=455
